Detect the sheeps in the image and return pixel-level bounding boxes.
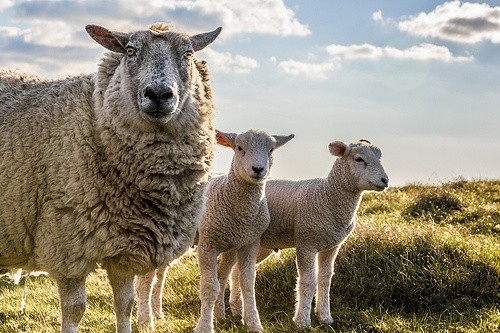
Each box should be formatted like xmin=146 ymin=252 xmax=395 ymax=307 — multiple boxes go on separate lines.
xmin=0 ymin=24 xmax=223 ymax=332
xmin=213 ymin=139 xmax=388 ymax=326
xmin=136 ymin=128 xmax=295 ymax=333
